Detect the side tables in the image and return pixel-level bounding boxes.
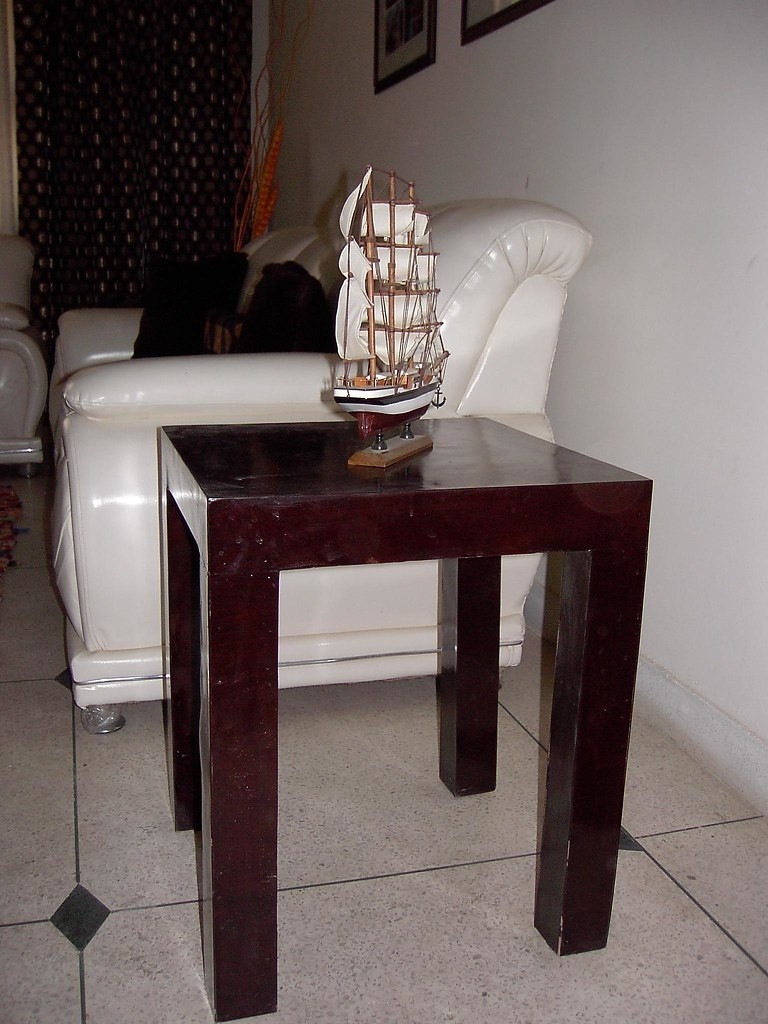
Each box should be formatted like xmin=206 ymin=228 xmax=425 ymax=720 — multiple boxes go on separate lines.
xmin=155 ymin=415 xmax=654 ymax=1024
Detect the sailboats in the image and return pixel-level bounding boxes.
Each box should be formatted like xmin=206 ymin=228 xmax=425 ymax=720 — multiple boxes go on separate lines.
xmin=333 ymin=165 xmax=451 ymax=468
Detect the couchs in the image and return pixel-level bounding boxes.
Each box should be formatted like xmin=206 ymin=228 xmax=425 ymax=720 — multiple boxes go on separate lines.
xmin=0 ymin=233 xmax=48 ymax=477
xmin=48 ymin=195 xmax=592 ymax=734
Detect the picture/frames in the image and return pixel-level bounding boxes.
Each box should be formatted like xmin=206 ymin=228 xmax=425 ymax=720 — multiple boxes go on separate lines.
xmin=460 ymin=0 xmax=555 ymax=47
xmin=373 ymin=0 xmax=437 ymax=95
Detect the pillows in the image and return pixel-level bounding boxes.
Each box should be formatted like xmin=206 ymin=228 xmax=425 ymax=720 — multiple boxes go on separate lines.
xmin=131 ymin=248 xmax=249 ymax=359
xmin=227 ymin=260 xmax=336 ymax=355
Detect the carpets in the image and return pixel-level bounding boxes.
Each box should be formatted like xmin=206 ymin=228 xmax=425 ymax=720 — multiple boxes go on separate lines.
xmin=0 ymin=485 xmax=31 ymax=578
